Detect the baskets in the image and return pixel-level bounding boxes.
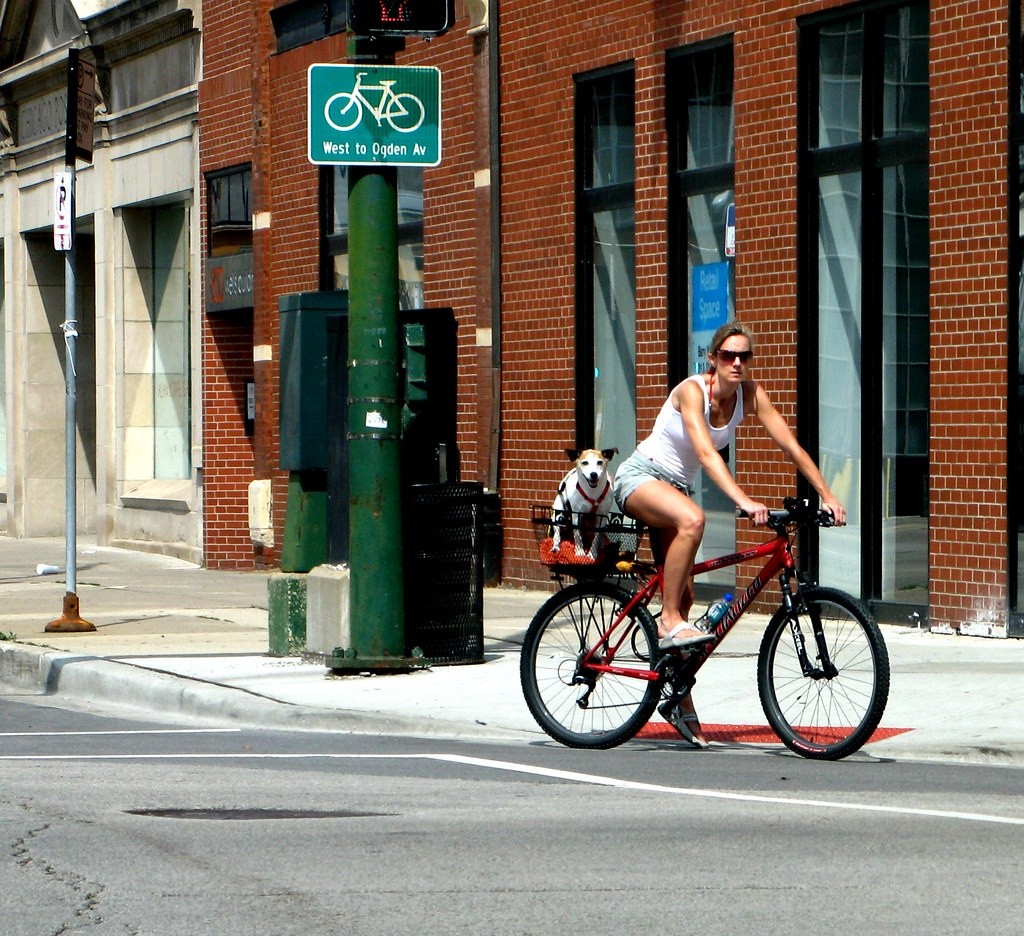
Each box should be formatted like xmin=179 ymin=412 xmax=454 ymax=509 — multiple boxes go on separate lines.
xmin=531 ymin=504 xmax=643 ymax=572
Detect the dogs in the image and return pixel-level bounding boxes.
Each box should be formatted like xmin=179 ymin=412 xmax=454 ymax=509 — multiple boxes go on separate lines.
xmin=548 ymin=446 xmax=619 ymax=561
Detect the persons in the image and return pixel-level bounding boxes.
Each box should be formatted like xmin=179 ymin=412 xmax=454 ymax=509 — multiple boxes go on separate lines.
xmin=617 ymin=324 xmax=845 ymax=748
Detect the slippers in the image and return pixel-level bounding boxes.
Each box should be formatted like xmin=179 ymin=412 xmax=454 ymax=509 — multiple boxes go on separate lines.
xmin=658 ymin=699 xmax=710 ymax=748
xmin=658 ymin=621 xmax=716 ymax=648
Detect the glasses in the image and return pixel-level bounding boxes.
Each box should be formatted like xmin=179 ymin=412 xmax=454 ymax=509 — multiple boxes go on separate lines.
xmin=717 ymin=349 xmax=753 ymax=362
